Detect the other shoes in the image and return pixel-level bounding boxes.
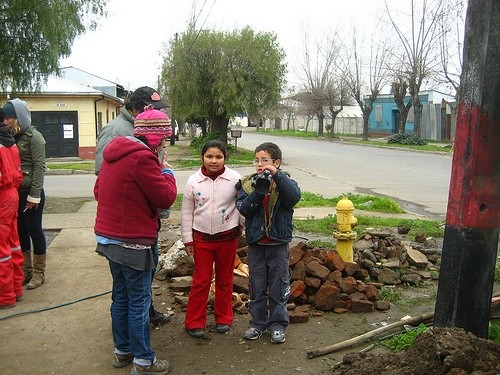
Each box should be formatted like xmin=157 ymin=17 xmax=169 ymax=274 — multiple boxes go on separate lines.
xmin=130 ymin=358 xmax=174 ymax=375
xmin=16 ymin=295 xmax=25 ymax=303
xmin=0 ymin=303 xmax=18 ymax=309
xmin=112 ymin=353 xmax=134 ymax=368
xmin=149 ymin=310 xmax=172 ymax=324
xmin=187 ymin=329 xmax=205 ymax=338
xmin=216 ymin=324 xmax=232 ymax=333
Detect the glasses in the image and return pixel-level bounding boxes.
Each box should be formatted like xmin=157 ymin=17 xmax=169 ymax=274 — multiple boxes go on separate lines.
xmin=252 ymin=158 xmax=276 ymax=165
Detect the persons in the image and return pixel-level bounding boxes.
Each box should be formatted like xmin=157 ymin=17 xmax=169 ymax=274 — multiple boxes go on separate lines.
xmin=235 ymin=142 xmax=301 ymax=344
xmin=181 ymin=140 xmax=245 ymax=338
xmin=1 ymin=98 xmax=47 ymax=289
xmin=94 ymin=105 xmax=177 ymax=375
xmin=0 ymin=107 xmax=25 ymax=310
xmin=95 ymin=86 xmax=171 ymax=329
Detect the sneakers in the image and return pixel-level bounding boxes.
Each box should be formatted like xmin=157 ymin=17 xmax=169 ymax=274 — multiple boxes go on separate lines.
xmin=243 ymin=327 xmax=268 ymax=340
xmin=271 ymin=330 xmax=286 ymax=343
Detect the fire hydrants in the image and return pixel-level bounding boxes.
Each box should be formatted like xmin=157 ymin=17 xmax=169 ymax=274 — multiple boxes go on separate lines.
xmin=332 ymin=194 xmax=359 ymax=263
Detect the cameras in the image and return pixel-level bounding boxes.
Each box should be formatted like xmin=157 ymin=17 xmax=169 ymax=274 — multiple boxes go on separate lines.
xmin=256 ymin=170 xmax=272 ymax=195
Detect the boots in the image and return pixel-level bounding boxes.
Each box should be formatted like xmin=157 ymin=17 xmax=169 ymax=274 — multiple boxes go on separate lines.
xmin=21 ymin=250 xmax=33 ymax=286
xmin=25 ymin=252 xmax=48 ymax=290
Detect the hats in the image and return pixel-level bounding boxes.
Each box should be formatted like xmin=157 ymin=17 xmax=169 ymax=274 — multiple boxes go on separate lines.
xmin=2 ymin=101 xmax=18 ymax=118
xmin=129 ymin=85 xmax=170 ymax=109
xmin=133 ymin=104 xmax=174 ymax=153
